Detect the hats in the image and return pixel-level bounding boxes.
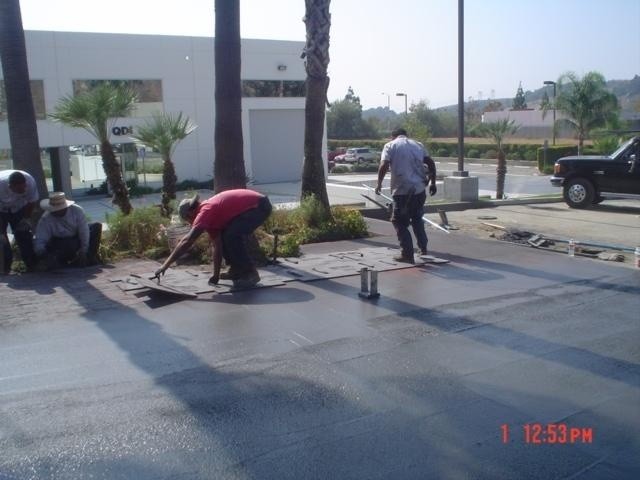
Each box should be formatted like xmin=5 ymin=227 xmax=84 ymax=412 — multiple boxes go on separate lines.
xmin=178 ymin=194 xmax=202 ymax=220
xmin=39 ymin=192 xmax=75 ymax=213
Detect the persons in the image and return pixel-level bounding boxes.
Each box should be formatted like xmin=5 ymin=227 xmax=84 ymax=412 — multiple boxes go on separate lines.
xmin=32 ymin=191 xmax=103 ymax=271
xmin=1 ymin=169 xmax=37 ymax=274
xmin=154 ymin=188 xmax=273 ymax=293
xmin=376 ymin=128 xmax=438 ymax=265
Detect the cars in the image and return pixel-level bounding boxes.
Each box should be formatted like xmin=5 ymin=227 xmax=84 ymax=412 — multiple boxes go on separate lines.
xmin=327 ymin=146 xmax=379 ymax=164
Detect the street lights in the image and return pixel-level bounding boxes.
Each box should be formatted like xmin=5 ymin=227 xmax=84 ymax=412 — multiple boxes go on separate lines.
xmin=397 ymin=93 xmax=408 ymax=116
xmin=543 ymin=81 xmax=557 ymax=147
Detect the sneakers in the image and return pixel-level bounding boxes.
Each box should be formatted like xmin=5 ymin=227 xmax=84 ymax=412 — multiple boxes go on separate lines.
xmin=394 ymin=256 xmax=414 ymax=264
xmin=417 ymin=249 xmax=427 ymax=255
xmin=219 ymin=271 xmax=239 ymax=280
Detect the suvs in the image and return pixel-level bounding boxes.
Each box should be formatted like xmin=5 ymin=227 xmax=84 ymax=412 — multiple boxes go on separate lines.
xmin=550 ymin=134 xmax=640 ymax=209
